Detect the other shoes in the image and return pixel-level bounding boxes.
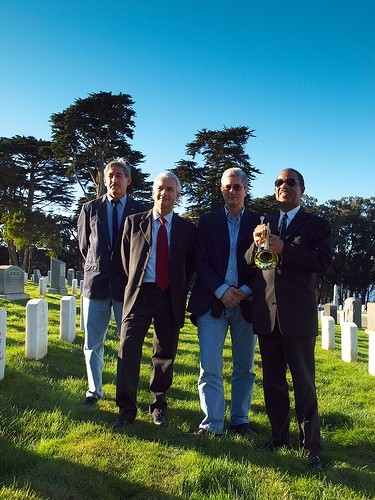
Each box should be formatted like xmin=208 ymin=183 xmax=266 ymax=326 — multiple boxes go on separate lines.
xmin=83 ymin=397 xmax=98 ymax=405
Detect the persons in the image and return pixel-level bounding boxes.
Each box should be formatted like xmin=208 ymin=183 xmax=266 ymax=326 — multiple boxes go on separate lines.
xmin=187 ymin=167 xmax=266 ymax=439
xmin=111 ymin=171 xmax=196 ymax=432
xmin=240 ymin=168 xmax=333 ymax=471
xmin=78 ymin=160 xmax=150 ymax=406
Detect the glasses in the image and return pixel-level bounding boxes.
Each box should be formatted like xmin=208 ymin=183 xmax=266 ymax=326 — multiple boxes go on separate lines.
xmin=275 ymin=177 xmax=299 ymax=187
xmin=223 ymin=185 xmax=243 ymax=191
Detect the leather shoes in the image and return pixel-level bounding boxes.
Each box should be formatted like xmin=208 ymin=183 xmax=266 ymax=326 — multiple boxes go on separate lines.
xmin=264 ymin=439 xmax=290 ymax=451
xmin=111 ymin=413 xmax=136 ymax=429
xmin=149 ymin=414 xmax=167 ymax=426
xmin=231 ymin=423 xmax=259 ymax=438
xmin=308 ymin=456 xmax=323 ymax=471
xmin=182 ymin=428 xmax=221 ymax=438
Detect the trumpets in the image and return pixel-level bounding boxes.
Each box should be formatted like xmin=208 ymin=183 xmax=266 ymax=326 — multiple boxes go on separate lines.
xmin=253 ymin=216 xmax=280 ymax=272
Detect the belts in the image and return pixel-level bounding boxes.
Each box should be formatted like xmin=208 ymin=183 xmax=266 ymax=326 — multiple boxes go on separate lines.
xmin=142 ymin=283 xmax=156 ymax=286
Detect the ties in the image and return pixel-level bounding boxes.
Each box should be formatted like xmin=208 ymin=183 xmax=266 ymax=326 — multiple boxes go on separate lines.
xmin=277 ymin=214 xmax=288 ymax=263
xmin=156 ymin=217 xmax=169 ymax=290
xmin=111 ymin=199 xmax=121 ymax=251
